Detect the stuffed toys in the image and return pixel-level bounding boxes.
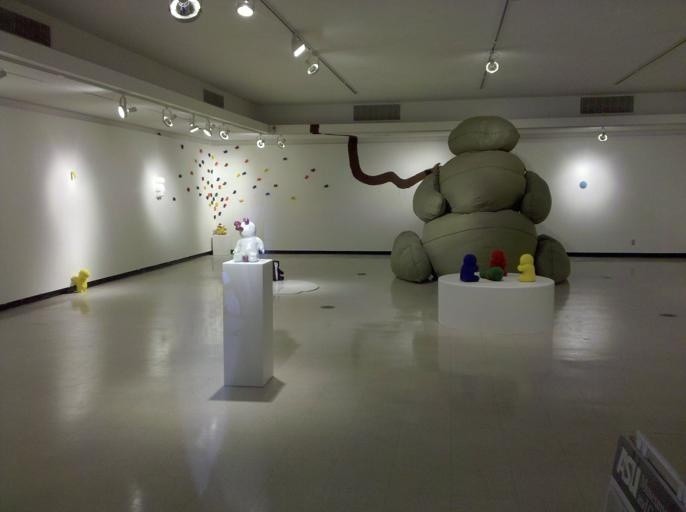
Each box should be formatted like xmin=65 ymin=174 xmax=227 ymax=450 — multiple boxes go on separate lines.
xmin=391 ymin=117 xmax=573 ymax=284
xmin=72 ymin=270 xmax=90 ymax=293
xmin=273 ymin=261 xmax=284 ymax=281
xmin=232 ymin=218 xmax=265 ymax=262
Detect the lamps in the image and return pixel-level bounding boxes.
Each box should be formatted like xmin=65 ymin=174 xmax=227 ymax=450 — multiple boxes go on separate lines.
xmin=116 ymin=94 xmax=231 ymax=144
xmin=168 ymin=0 xmax=201 ymax=23
xmin=290 ymin=36 xmax=319 ymax=76
